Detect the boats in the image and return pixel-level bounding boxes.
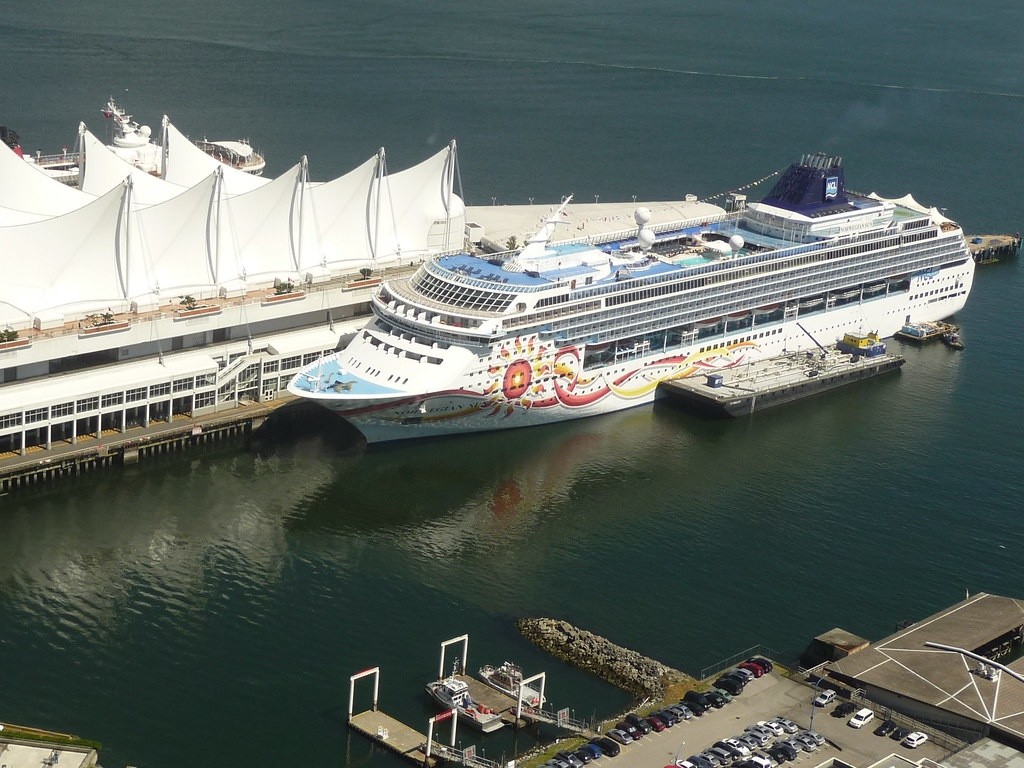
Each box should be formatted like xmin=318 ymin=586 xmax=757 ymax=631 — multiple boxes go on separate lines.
xmin=585 ymin=342 xmax=610 ymax=356
xmin=617 ymin=337 xmax=637 ymax=347
xmin=658 ymin=329 xmax=905 ymax=419
xmin=696 ymin=317 xmax=720 ymax=329
xmin=19 ymin=92 xmax=266 ymax=190
xmin=941 ymin=332 xmax=966 ymax=350
xmin=890 ymin=273 xmax=910 ymax=283
xmin=834 ymin=289 xmax=860 ymax=300
xmin=425 ymin=655 xmax=506 ymax=734
xmin=799 ymin=296 xmax=823 ymax=309
xmin=893 ymin=321 xmax=959 ymax=344
xmin=749 ymin=304 xmax=780 ymax=316
xmin=863 ymin=282 xmax=886 ymax=295
xmin=477 ymin=661 xmax=548 ymax=709
xmin=726 ymin=309 xmax=751 ymax=323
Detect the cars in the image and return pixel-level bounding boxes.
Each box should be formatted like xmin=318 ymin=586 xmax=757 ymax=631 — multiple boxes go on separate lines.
xmin=873 ymin=720 xmax=897 ymax=736
xmin=890 ymin=727 xmax=910 ymax=740
xmin=739 ymin=662 xmax=764 ymax=678
xmin=733 ymin=668 xmax=754 ymax=681
xmin=746 ymin=657 xmax=773 ymax=673
xmin=834 ymin=701 xmax=857 ymax=718
xmin=533 ymin=672 xmax=825 ymax=768
xmin=904 ymin=731 xmax=930 ymax=748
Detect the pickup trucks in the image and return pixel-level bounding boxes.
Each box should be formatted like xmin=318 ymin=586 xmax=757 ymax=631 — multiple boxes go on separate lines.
xmin=814 ymin=689 xmax=838 ymax=708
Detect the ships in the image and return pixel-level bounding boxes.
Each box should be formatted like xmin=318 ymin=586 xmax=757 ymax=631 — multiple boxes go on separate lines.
xmin=285 ymin=151 xmax=975 ymax=446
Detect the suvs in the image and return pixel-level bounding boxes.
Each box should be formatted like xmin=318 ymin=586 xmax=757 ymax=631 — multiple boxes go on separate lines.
xmin=847 ymin=708 xmax=875 ymax=728
